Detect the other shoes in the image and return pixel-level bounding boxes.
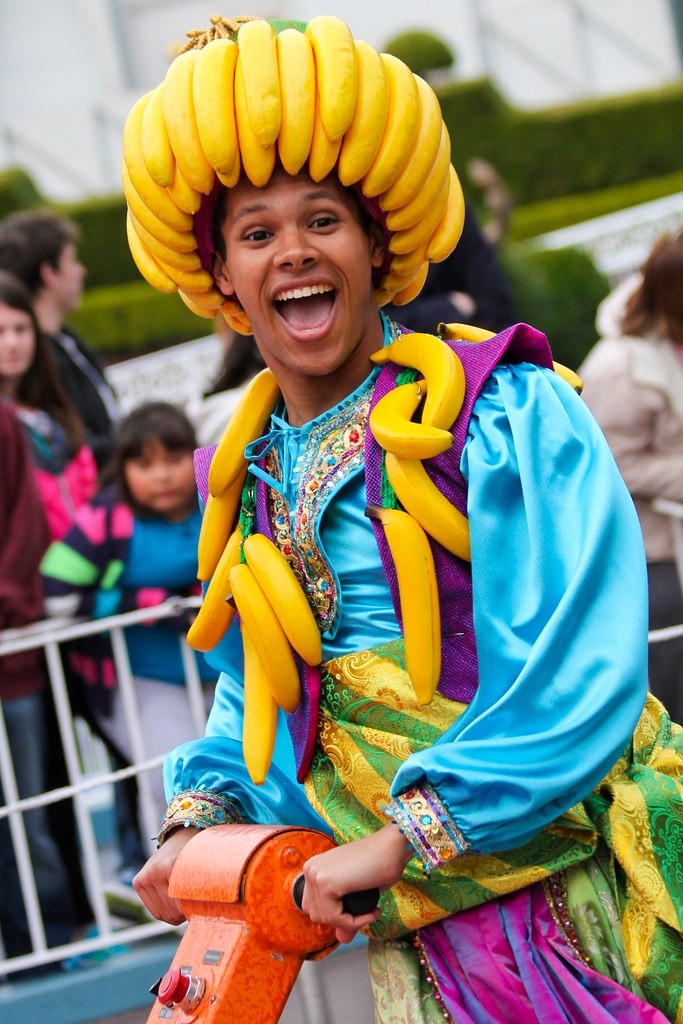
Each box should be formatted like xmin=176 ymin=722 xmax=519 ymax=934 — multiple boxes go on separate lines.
xmin=103 ymin=865 xmax=153 ymax=923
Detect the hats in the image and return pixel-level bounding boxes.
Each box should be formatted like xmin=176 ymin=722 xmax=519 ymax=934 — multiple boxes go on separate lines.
xmin=120 ymin=14 xmax=465 ymax=337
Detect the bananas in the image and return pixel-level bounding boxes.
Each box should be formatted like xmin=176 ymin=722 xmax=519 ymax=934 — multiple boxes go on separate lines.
xmin=365 ymin=323 xmax=581 ymax=707
xmin=120 ymin=15 xmax=466 ymax=337
xmin=186 ymin=368 xmax=322 ymax=789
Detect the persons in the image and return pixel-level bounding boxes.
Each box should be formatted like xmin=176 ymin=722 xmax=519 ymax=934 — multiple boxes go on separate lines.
xmin=569 ymin=226 xmax=683 ymax=724
xmin=0 ymin=275 xmax=123 ymax=980
xmin=121 ymin=16 xmax=683 ymax=1024
xmin=41 ymin=400 xmax=226 ymax=853
xmin=384 ymin=199 xmax=501 ymax=344
xmin=0 ymin=212 xmax=163 ymax=922
